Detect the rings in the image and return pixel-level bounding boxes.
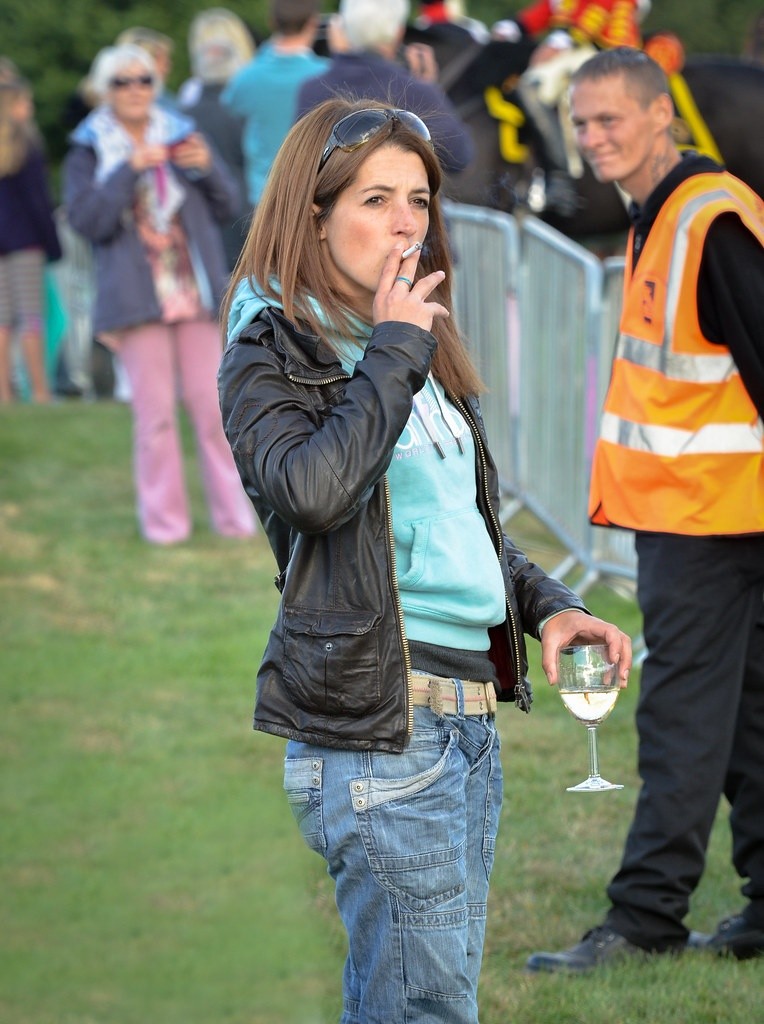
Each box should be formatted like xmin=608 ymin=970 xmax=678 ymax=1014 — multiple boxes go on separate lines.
xmin=395 ymin=277 xmax=412 ymax=290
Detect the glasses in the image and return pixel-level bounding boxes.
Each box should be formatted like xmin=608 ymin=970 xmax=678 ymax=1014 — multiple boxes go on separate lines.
xmin=317 ymin=108 xmax=434 ymax=176
xmin=111 ymin=75 xmax=151 ymax=90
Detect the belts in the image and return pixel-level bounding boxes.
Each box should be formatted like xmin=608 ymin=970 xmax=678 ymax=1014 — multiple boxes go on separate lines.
xmin=410 ymin=671 xmax=498 ymax=721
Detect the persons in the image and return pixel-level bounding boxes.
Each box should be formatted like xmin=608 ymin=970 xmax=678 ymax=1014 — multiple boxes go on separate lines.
xmin=528 ymin=48 xmax=763 ymax=974
xmin=61 ymin=47 xmax=255 ymax=542
xmin=0 ymin=0 xmax=626 ymax=402
xmin=217 ymin=101 xmax=632 ymax=1023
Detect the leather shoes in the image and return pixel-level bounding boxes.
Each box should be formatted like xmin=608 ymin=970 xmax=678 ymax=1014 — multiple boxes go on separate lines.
xmin=525 ymin=924 xmax=638 ymax=974
xmin=687 ymin=912 xmax=764 ymax=961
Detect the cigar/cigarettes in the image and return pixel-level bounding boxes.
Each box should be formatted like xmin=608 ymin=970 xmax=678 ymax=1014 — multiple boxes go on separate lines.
xmin=398 ymin=239 xmax=422 ymax=263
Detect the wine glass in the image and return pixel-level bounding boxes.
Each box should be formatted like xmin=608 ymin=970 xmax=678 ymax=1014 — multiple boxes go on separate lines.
xmin=556 ymin=644 xmax=625 ymax=793
